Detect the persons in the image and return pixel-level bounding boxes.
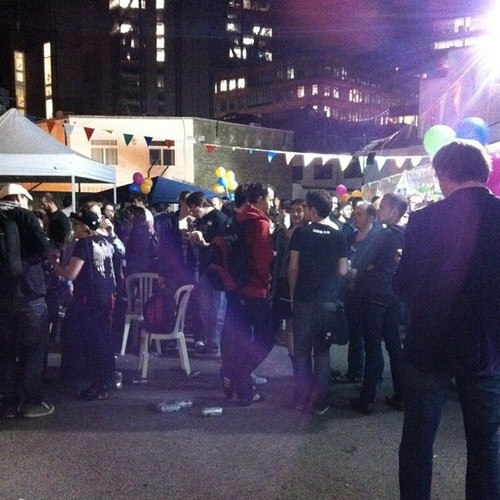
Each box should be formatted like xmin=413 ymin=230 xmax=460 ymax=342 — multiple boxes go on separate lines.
xmin=390 ymin=142 xmax=500 ymax=500
xmin=0 ymin=180 xmax=411 ymax=422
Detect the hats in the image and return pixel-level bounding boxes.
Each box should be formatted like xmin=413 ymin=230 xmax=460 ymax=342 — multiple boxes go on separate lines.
xmin=0 ymin=184 xmax=33 ymax=200
xmin=70 ymin=209 xmax=99 ymax=230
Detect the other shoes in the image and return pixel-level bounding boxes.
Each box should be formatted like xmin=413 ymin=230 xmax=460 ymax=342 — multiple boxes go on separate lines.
xmin=295 ymin=400 xmax=304 ymax=410
xmin=388 ymin=399 xmax=405 ymax=411
xmin=197 ymin=340 xmax=209 ymax=354
xmin=5 ymin=403 xmax=21 ymax=418
xmin=249 ymin=374 xmax=268 ymax=384
xmin=315 ymin=399 xmax=329 ymax=415
xmin=219 ymin=367 xmax=234 ymax=402
xmin=352 ymin=400 xmax=375 ymax=414
xmin=26 ymin=403 xmax=55 ymax=417
xmin=335 ymin=365 xmax=363 ymax=382
xmin=241 ymin=392 xmax=265 ymax=406
xmin=253 ymin=386 xmax=257 ymax=391
xmin=80 ymin=383 xmax=109 ymax=400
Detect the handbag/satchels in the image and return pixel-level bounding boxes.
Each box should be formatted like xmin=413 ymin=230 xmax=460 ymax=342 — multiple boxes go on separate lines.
xmin=325 ymin=307 xmax=349 ymax=345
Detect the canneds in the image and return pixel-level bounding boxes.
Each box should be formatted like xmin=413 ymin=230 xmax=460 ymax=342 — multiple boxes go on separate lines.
xmin=131 ymin=378 xmax=151 ymax=385
xmin=113 ymin=371 xmax=123 ymax=389
xmin=201 ymin=406 xmax=223 ymax=417
xmin=173 ymin=399 xmax=193 ymax=408
xmin=190 ymin=370 xmax=202 ymax=378
xmin=155 ymin=402 xmax=181 ymax=413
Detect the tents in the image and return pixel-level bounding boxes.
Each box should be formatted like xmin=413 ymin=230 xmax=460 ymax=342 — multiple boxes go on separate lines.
xmin=0 ymin=106 xmax=117 ymax=186
xmin=62 ymin=175 xmax=228 ymax=207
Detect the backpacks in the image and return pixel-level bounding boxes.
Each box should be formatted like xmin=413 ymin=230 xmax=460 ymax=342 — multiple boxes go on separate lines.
xmin=143 ymin=290 xmax=178 ymax=333
xmin=208 ymin=217 xmax=249 ymax=292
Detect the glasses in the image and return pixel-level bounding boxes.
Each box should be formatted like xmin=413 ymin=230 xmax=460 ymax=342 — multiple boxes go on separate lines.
xmin=291 ymin=208 xmax=304 ymax=215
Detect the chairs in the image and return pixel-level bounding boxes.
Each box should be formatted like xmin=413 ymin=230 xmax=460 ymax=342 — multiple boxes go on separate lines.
xmin=120 ymin=274 xmax=162 ymax=356
xmin=137 ymin=284 xmax=195 ymax=384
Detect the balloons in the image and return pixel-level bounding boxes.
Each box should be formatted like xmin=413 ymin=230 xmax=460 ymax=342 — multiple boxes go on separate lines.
xmin=422 ymin=116 xmax=500 ymax=196
xmin=129 ymin=171 xmax=154 ymax=196
xmin=210 ymin=165 xmax=239 ymax=195
xmin=336 ymin=183 xmax=362 ymax=203
